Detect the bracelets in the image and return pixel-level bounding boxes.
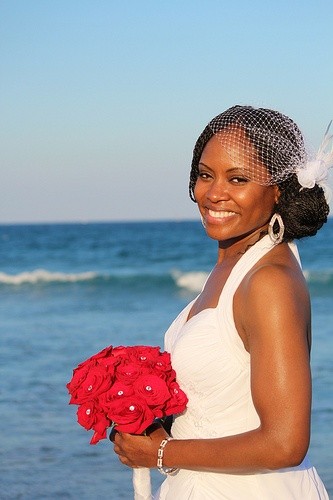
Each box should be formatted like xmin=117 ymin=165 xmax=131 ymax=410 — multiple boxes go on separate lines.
xmin=156 ymin=436 xmax=177 ymax=475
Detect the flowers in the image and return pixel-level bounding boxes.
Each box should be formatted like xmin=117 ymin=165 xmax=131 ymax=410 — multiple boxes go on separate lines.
xmin=64 ymin=344 xmax=189 ymax=500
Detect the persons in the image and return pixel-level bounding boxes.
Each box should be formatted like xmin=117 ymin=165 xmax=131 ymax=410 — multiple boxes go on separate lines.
xmin=111 ymin=105 xmax=333 ymax=500
